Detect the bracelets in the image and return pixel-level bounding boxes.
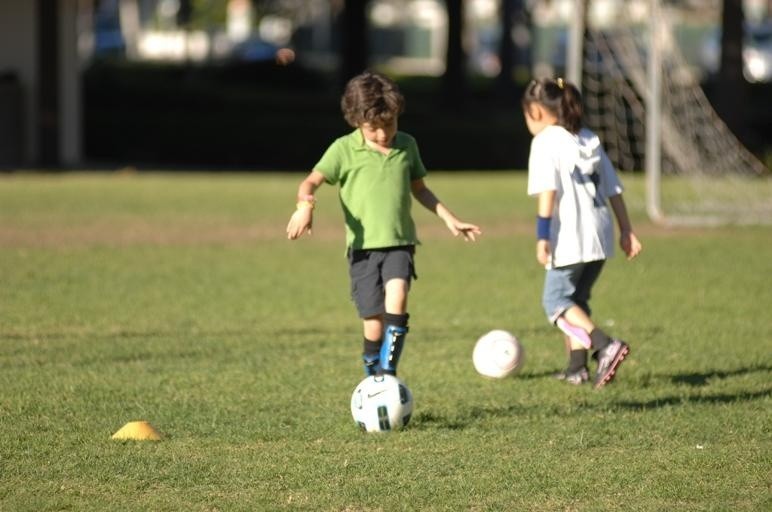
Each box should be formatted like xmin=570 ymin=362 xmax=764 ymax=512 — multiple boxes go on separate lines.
xmin=535 ymin=216 xmax=552 ymax=240
xmin=294 ymin=194 xmax=316 ymax=207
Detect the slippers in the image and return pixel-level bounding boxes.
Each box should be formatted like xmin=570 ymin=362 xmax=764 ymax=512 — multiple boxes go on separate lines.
xmin=590 ymin=338 xmax=630 ymax=390
xmin=552 ymin=366 xmax=590 ymax=386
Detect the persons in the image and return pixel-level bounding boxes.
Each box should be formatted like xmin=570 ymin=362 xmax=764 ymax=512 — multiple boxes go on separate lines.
xmin=285 ymin=73 xmax=483 ymax=375
xmin=242 ymin=13 xmax=299 ymax=66
xmin=518 ymin=78 xmax=643 ymax=388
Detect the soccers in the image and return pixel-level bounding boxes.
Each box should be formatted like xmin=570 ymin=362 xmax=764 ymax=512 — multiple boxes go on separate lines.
xmin=472 ymin=330 xmax=524 ymax=378
xmin=351 ymin=374 xmax=413 ymax=433
